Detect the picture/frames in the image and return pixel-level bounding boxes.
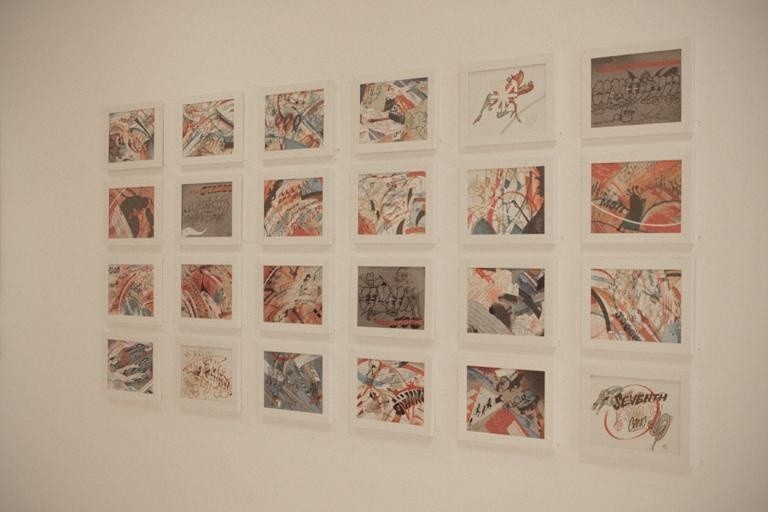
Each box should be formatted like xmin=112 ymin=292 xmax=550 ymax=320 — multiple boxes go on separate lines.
xmin=576 ymin=39 xmax=695 ymax=459
xmin=457 ymin=53 xmax=560 ymax=447
xmin=99 ymin=88 xmax=245 ymax=418
xmin=257 ymin=69 xmax=436 ymax=439
